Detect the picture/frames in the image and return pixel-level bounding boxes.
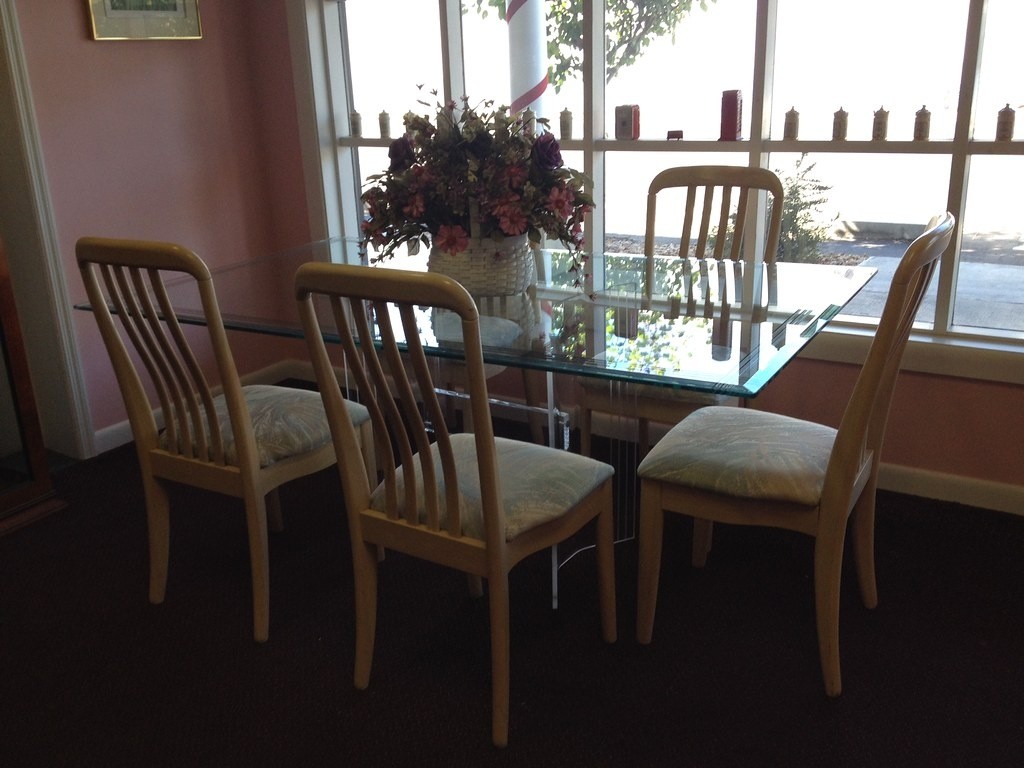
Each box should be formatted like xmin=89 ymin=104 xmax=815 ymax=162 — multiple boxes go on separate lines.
xmin=86 ymin=0 xmax=203 ymax=40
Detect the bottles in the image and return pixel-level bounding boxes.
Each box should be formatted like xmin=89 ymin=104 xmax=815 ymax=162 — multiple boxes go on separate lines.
xmin=379 ymin=110 xmax=391 ymax=139
xmin=871 ymin=105 xmax=890 ymax=142
xmin=522 ymin=108 xmax=536 ymax=139
xmin=783 ymin=106 xmax=799 ymax=141
xmin=349 ymin=110 xmax=362 ymax=138
xmin=995 ymin=102 xmax=1015 ymax=141
xmin=560 ymin=107 xmax=573 ymax=141
xmin=832 ymin=106 xmax=849 ymax=141
xmin=913 ymin=105 xmax=931 ymax=142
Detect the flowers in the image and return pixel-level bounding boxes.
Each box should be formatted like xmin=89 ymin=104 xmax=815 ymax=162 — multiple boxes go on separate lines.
xmin=358 ymin=83 xmax=597 ymax=303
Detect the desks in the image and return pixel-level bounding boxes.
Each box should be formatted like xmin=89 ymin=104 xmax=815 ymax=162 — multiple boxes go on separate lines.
xmin=73 ymin=235 xmax=880 ymax=610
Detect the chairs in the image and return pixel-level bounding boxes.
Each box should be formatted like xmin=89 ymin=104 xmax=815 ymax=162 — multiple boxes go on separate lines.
xmin=439 ymin=232 xmax=546 ymax=446
xmin=636 ymin=214 xmax=957 ymax=695
xmin=293 ymin=260 xmax=617 ymax=746
xmin=580 ymin=164 xmax=785 ymax=565
xmin=75 ymin=237 xmax=386 ymax=646
